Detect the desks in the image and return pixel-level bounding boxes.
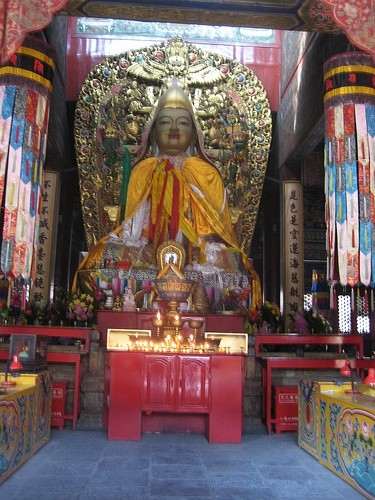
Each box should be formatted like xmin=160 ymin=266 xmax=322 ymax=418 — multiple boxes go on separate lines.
xmin=258 ymin=356 xmax=375 ymax=434
xmin=97 ymin=310 xmax=246 ymax=347
xmin=104 ymin=350 xmax=246 ymax=443
xmin=0 ymin=325 xmax=92 ymax=353
xmin=0 ymin=350 xmax=83 ymax=429
xmin=298 ymin=379 xmax=375 ymax=500
xmin=255 ymin=332 xmax=364 ymax=358
xmin=0 ymin=370 xmax=52 ymax=485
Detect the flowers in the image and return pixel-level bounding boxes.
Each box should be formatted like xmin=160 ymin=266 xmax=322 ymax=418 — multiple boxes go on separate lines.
xmin=65 ymin=292 xmax=95 ymax=322
xmin=0 ymin=291 xmax=54 ymax=322
xmin=221 ymin=286 xmax=333 ymax=333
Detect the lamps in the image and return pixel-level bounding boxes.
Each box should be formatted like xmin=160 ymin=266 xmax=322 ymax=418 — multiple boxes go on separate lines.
xmin=364 ymin=368 xmax=375 ymax=383
xmin=341 ymin=359 xmax=359 ymax=393
xmin=4 ymin=355 xmax=23 ymax=385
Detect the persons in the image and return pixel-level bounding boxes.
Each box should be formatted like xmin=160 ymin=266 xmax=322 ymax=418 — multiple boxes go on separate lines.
xmin=72 ymin=76 xmax=264 ymax=312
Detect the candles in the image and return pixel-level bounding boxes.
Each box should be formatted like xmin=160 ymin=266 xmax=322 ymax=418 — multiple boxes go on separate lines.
xmin=7 ymin=286 xmax=13 ymax=307
